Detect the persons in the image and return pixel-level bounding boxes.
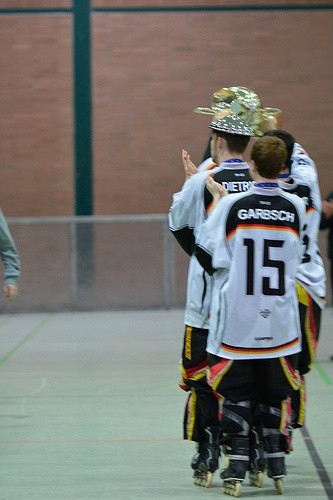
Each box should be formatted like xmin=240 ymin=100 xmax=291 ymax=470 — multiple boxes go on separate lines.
xmin=262 ymin=129 xmax=328 ymax=455
xmin=168 ymin=111 xmax=263 ymax=474
xmin=0 ymin=206 xmax=21 ymax=300
xmin=193 ymin=135 xmax=303 ymax=481
xmin=194 ymin=85 xmax=281 ymax=166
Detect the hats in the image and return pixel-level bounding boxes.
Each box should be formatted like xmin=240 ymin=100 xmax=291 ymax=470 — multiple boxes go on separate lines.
xmin=193 ymin=86 xmax=260 ymax=116
xmin=259 ymin=107 xmax=282 ymax=130
xmin=207 ymin=112 xmax=262 ymax=137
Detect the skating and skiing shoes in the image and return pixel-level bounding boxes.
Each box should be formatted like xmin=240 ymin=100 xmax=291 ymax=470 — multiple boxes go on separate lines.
xmin=190 ymin=443 xmax=220 ymax=487
xmin=266 ymin=457 xmax=287 ymax=495
xmin=246 ymin=448 xmax=264 ymax=488
xmin=219 ymin=459 xmax=249 ymax=497
